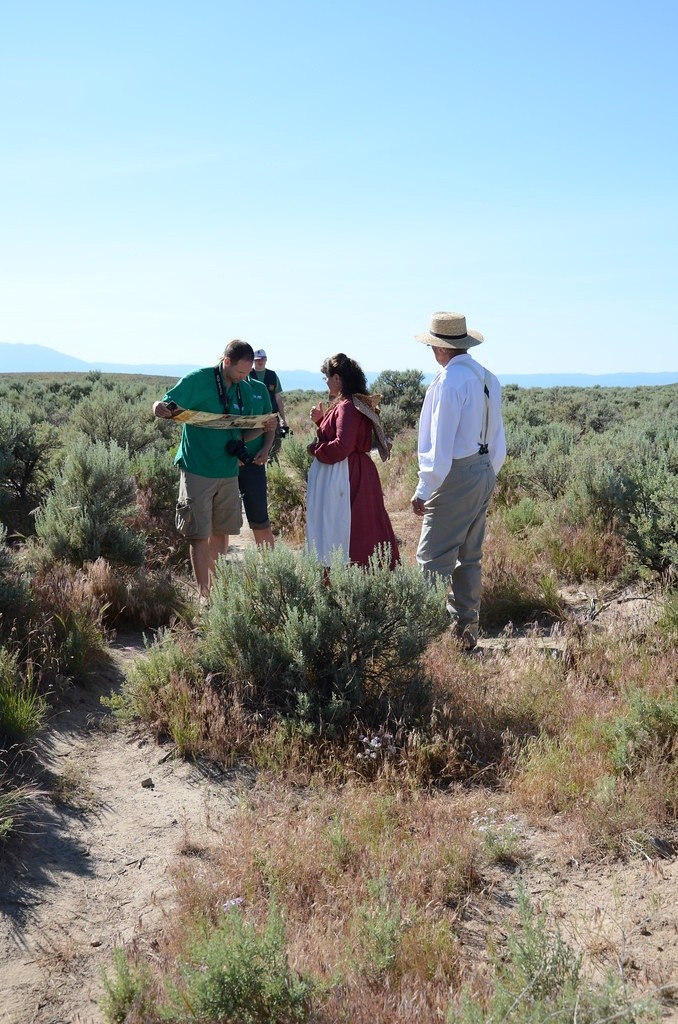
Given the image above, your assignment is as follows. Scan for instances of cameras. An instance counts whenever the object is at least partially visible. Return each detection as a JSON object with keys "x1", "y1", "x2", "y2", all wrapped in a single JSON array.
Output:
[{"x1": 227, "y1": 440, "x2": 254, "y2": 465}]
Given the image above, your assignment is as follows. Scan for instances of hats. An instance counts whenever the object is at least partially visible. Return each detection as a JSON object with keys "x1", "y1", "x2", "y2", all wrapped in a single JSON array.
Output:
[
  {"x1": 254, "y1": 349, "x2": 266, "y2": 360},
  {"x1": 415, "y1": 312, "x2": 484, "y2": 349}
]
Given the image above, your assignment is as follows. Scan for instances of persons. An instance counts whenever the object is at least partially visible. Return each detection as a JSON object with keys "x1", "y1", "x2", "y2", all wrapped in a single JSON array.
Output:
[
  {"x1": 152, "y1": 339, "x2": 279, "y2": 608},
  {"x1": 248, "y1": 349, "x2": 287, "y2": 469},
  {"x1": 305, "y1": 353, "x2": 401, "y2": 593},
  {"x1": 409, "y1": 312, "x2": 506, "y2": 652},
  {"x1": 218, "y1": 353, "x2": 275, "y2": 552}
]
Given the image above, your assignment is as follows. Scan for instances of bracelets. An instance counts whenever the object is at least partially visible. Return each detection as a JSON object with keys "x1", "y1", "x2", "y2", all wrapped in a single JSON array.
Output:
[{"x1": 262, "y1": 427, "x2": 267, "y2": 432}]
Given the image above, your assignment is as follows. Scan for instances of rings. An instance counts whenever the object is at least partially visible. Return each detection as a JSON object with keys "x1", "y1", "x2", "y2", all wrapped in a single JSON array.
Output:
[
  {"x1": 261, "y1": 462, "x2": 263, "y2": 465},
  {"x1": 417, "y1": 509, "x2": 420, "y2": 511}
]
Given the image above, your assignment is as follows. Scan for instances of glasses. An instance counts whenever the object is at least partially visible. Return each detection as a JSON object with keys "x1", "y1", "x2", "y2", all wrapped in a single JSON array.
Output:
[
  {"x1": 427, "y1": 344, "x2": 433, "y2": 350},
  {"x1": 322, "y1": 376, "x2": 332, "y2": 382}
]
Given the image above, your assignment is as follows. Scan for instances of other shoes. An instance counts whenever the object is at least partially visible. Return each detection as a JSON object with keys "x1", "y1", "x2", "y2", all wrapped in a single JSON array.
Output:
[{"x1": 451, "y1": 632, "x2": 478, "y2": 650}]
